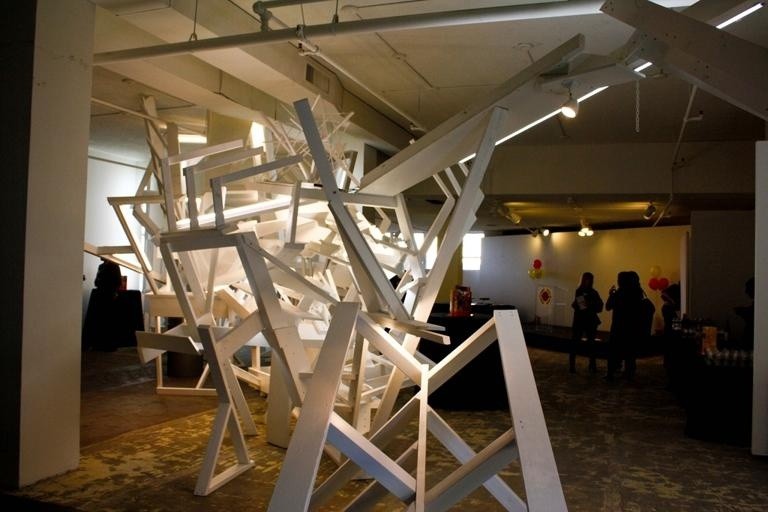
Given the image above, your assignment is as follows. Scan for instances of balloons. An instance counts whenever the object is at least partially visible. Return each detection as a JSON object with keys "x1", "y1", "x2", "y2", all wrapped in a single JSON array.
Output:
[
  {"x1": 532, "y1": 269, "x2": 541, "y2": 279},
  {"x1": 533, "y1": 259, "x2": 541, "y2": 270},
  {"x1": 651, "y1": 264, "x2": 665, "y2": 276},
  {"x1": 528, "y1": 268, "x2": 535, "y2": 279}
]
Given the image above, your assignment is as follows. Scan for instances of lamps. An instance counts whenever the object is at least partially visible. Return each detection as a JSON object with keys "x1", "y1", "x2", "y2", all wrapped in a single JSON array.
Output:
[
  {"x1": 494, "y1": 197, "x2": 657, "y2": 239},
  {"x1": 551, "y1": 77, "x2": 582, "y2": 123}
]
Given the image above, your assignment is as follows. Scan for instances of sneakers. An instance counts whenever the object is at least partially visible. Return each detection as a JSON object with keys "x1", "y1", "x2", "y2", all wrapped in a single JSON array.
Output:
[{"x1": 568, "y1": 367, "x2": 635, "y2": 385}]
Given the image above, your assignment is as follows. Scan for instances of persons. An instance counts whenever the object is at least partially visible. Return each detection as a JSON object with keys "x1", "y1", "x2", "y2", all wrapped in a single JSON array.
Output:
[
  {"x1": 569, "y1": 270, "x2": 682, "y2": 380},
  {"x1": 87, "y1": 252, "x2": 122, "y2": 353},
  {"x1": 734, "y1": 275, "x2": 754, "y2": 350}
]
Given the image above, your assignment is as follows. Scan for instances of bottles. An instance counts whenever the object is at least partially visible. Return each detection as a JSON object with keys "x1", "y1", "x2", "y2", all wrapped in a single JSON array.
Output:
[{"x1": 450, "y1": 285, "x2": 472, "y2": 316}]
